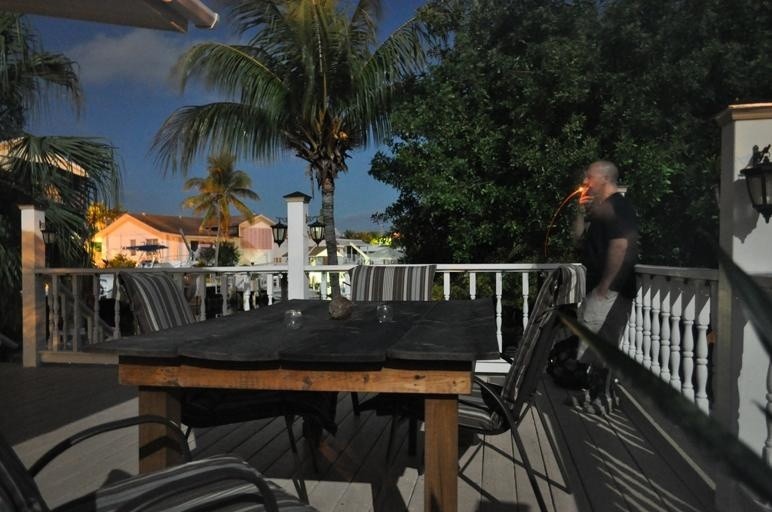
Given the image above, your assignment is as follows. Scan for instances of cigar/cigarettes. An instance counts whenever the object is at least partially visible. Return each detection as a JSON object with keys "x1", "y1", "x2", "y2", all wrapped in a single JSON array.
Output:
[{"x1": 579, "y1": 188, "x2": 587, "y2": 191}]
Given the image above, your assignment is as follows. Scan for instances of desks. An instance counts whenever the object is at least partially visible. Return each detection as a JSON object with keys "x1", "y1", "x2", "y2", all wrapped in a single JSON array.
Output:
[{"x1": 82, "y1": 300, "x2": 498, "y2": 511}]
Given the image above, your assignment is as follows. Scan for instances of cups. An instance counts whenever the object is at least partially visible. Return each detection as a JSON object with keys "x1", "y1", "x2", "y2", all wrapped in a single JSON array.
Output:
[
  {"x1": 284, "y1": 309, "x2": 304, "y2": 329},
  {"x1": 376, "y1": 305, "x2": 393, "y2": 324}
]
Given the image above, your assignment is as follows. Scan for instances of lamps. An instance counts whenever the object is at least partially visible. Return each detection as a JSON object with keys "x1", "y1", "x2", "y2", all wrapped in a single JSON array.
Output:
[
  {"x1": 739, "y1": 144, "x2": 772, "y2": 224},
  {"x1": 268, "y1": 213, "x2": 326, "y2": 249}
]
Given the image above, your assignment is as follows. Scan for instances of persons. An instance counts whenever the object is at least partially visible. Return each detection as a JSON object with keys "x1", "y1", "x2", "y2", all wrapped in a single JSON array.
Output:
[{"x1": 565, "y1": 161, "x2": 631, "y2": 410}]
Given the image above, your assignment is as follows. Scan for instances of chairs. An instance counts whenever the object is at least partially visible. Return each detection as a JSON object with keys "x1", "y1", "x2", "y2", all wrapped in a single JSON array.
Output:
[
  {"x1": 348, "y1": 265, "x2": 438, "y2": 417},
  {"x1": 357, "y1": 266, "x2": 571, "y2": 510},
  {"x1": 3, "y1": 415, "x2": 318, "y2": 511},
  {"x1": 117, "y1": 270, "x2": 340, "y2": 468}
]
